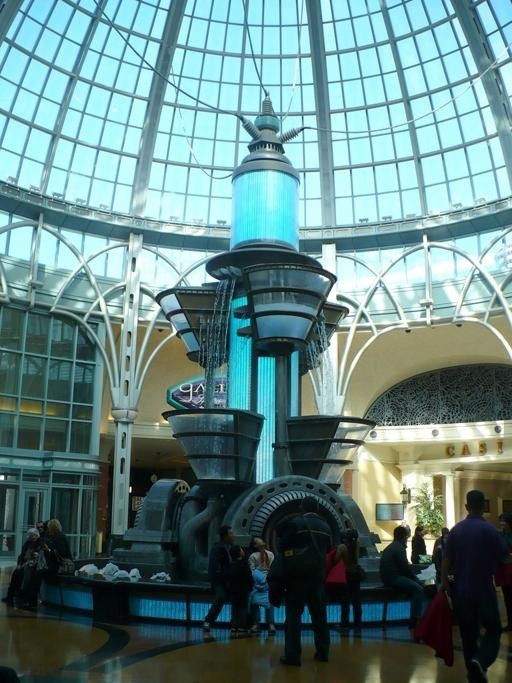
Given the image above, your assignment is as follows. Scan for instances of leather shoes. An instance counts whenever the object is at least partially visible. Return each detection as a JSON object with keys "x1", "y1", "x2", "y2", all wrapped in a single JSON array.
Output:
[
  {"x1": 280, "y1": 656, "x2": 301, "y2": 666},
  {"x1": 315, "y1": 653, "x2": 328, "y2": 662}
]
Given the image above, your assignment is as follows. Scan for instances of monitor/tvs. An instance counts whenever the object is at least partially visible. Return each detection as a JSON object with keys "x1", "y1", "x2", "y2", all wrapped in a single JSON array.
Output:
[{"x1": 376, "y1": 504, "x2": 404, "y2": 520}]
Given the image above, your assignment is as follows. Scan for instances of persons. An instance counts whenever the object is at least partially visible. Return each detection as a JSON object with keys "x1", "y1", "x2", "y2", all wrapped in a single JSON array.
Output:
[
  {"x1": 379, "y1": 526, "x2": 424, "y2": 631},
  {"x1": 411, "y1": 525, "x2": 426, "y2": 564},
  {"x1": 440, "y1": 488, "x2": 510, "y2": 683},
  {"x1": 432, "y1": 527, "x2": 449, "y2": 583},
  {"x1": 201, "y1": 524, "x2": 278, "y2": 634},
  {"x1": 1, "y1": 518, "x2": 73, "y2": 614},
  {"x1": 334, "y1": 528, "x2": 365, "y2": 628},
  {"x1": 493, "y1": 511, "x2": 512, "y2": 631},
  {"x1": 277, "y1": 495, "x2": 334, "y2": 666}
]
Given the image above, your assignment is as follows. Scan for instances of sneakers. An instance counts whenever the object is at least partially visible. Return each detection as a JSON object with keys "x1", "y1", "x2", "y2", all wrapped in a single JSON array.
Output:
[
  {"x1": 202, "y1": 622, "x2": 211, "y2": 631},
  {"x1": 469, "y1": 657, "x2": 489, "y2": 682},
  {"x1": 269, "y1": 623, "x2": 276, "y2": 632},
  {"x1": 2, "y1": 596, "x2": 14, "y2": 605},
  {"x1": 238, "y1": 623, "x2": 248, "y2": 632},
  {"x1": 250, "y1": 625, "x2": 260, "y2": 632},
  {"x1": 231, "y1": 624, "x2": 238, "y2": 631}
]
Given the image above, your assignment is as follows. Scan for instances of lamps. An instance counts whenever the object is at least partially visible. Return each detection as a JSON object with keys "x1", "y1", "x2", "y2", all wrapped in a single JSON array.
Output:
[{"x1": 399, "y1": 484, "x2": 411, "y2": 505}]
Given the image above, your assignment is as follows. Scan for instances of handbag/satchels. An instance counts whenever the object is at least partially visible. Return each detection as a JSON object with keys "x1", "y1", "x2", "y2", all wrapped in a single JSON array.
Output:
[{"x1": 287, "y1": 546, "x2": 324, "y2": 579}]
